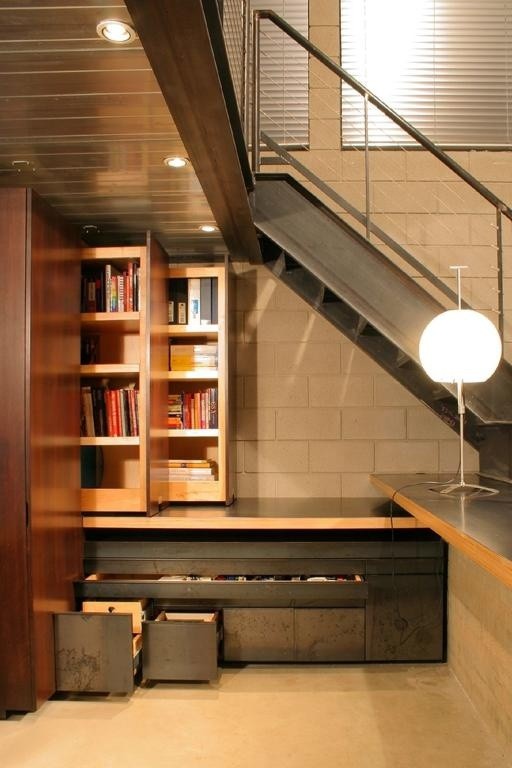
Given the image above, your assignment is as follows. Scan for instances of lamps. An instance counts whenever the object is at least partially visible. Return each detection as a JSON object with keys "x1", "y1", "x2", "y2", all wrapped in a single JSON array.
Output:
[{"x1": 417, "y1": 264, "x2": 505, "y2": 498}]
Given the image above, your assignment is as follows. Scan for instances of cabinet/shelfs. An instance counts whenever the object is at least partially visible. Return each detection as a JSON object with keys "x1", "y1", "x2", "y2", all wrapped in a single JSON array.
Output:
[
  {"x1": 0, "y1": 186, "x2": 84, "y2": 719},
  {"x1": 160, "y1": 250, "x2": 243, "y2": 507},
  {"x1": 38, "y1": 520, "x2": 447, "y2": 700},
  {"x1": 70, "y1": 228, "x2": 175, "y2": 515}
]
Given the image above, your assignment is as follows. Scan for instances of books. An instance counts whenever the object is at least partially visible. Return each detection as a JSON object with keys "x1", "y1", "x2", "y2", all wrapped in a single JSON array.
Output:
[
  {"x1": 167, "y1": 386, "x2": 217, "y2": 429},
  {"x1": 151, "y1": 459, "x2": 218, "y2": 481},
  {"x1": 81, "y1": 260, "x2": 141, "y2": 313},
  {"x1": 80, "y1": 378, "x2": 138, "y2": 436}
]
